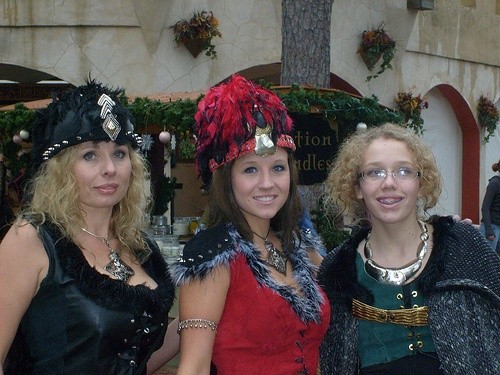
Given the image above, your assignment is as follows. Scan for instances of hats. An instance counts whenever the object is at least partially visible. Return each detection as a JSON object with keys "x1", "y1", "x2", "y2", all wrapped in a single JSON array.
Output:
[
  {"x1": 32, "y1": 70, "x2": 144, "y2": 171},
  {"x1": 191, "y1": 73, "x2": 296, "y2": 179}
]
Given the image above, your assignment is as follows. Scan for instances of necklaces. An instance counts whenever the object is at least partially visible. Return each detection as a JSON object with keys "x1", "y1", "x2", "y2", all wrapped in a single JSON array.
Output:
[
  {"x1": 80, "y1": 225, "x2": 135, "y2": 284},
  {"x1": 252, "y1": 228, "x2": 288, "y2": 277},
  {"x1": 364, "y1": 218, "x2": 430, "y2": 285}
]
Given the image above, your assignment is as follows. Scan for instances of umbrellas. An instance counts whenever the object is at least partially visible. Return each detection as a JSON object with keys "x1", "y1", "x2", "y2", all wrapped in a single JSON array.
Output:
[{"x1": 0, "y1": 98, "x2": 59, "y2": 110}]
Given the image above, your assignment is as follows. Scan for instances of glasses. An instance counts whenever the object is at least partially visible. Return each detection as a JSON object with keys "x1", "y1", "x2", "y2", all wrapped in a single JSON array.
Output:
[{"x1": 358, "y1": 168, "x2": 421, "y2": 182}]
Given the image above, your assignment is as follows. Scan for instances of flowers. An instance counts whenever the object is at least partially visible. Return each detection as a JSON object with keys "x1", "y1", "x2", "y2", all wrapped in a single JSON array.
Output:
[
  {"x1": 355, "y1": 21, "x2": 397, "y2": 81},
  {"x1": 476, "y1": 95, "x2": 500, "y2": 145},
  {"x1": 395, "y1": 89, "x2": 428, "y2": 139},
  {"x1": 171, "y1": 9, "x2": 223, "y2": 60}
]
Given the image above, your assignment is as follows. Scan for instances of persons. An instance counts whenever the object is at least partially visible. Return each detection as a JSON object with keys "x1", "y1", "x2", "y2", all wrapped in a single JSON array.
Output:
[
  {"x1": 315, "y1": 122, "x2": 500, "y2": 375},
  {"x1": 0, "y1": 123, "x2": 36, "y2": 243},
  {"x1": 480, "y1": 160, "x2": 500, "y2": 256},
  {"x1": 0, "y1": 66, "x2": 181, "y2": 375},
  {"x1": 176, "y1": 74, "x2": 330, "y2": 375},
  {"x1": 297, "y1": 188, "x2": 328, "y2": 266}
]
